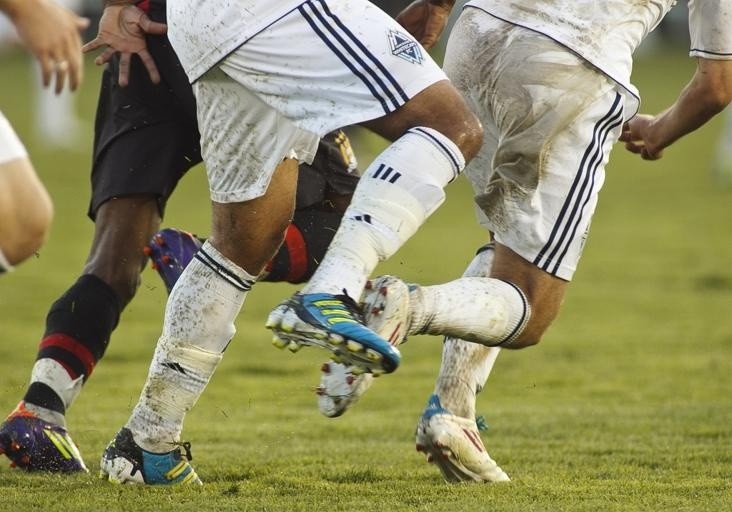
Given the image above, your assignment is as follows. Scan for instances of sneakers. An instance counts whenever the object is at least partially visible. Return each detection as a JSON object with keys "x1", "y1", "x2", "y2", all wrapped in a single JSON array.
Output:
[
  {"x1": 147, "y1": 227, "x2": 206, "y2": 293},
  {"x1": 316, "y1": 274, "x2": 413, "y2": 417},
  {"x1": 97, "y1": 428, "x2": 202, "y2": 487},
  {"x1": 266, "y1": 289, "x2": 402, "y2": 374},
  {"x1": 0, "y1": 404, "x2": 89, "y2": 472},
  {"x1": 415, "y1": 394, "x2": 511, "y2": 484}
]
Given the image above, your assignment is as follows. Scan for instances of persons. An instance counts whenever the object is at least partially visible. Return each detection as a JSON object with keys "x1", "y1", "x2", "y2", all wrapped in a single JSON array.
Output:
[
  {"x1": 1, "y1": 0, "x2": 90, "y2": 276},
  {"x1": 311, "y1": 2, "x2": 732, "y2": 483},
  {"x1": 1, "y1": 0, "x2": 454, "y2": 474},
  {"x1": 98, "y1": 0, "x2": 483, "y2": 490}
]
